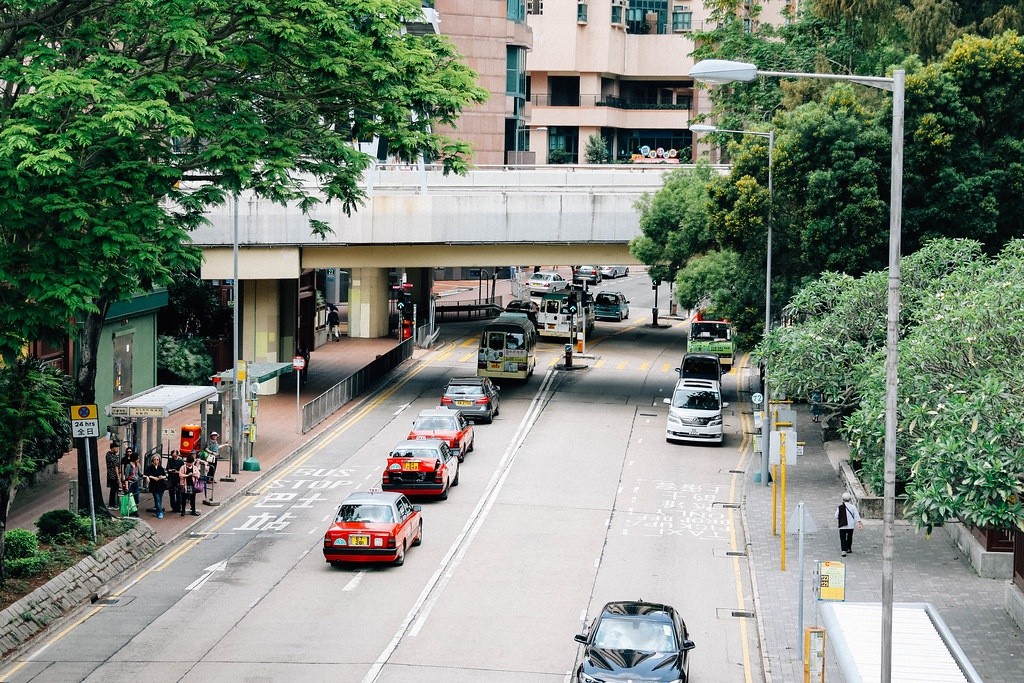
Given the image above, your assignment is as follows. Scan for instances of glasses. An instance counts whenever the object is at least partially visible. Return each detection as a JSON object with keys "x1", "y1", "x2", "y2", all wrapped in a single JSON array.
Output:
[
  {"x1": 212, "y1": 435, "x2": 217, "y2": 436},
  {"x1": 127, "y1": 451, "x2": 131, "y2": 452}
]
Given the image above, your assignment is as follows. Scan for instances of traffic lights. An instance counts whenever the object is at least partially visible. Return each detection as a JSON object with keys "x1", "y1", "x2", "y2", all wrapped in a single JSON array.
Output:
[
  {"x1": 397, "y1": 288, "x2": 404, "y2": 310},
  {"x1": 568, "y1": 292, "x2": 578, "y2": 314},
  {"x1": 581, "y1": 290, "x2": 592, "y2": 308},
  {"x1": 403, "y1": 291, "x2": 412, "y2": 306},
  {"x1": 651, "y1": 279, "x2": 657, "y2": 285}
]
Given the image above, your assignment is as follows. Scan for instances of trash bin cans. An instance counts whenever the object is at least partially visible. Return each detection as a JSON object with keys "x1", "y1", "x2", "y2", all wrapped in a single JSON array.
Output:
[
  {"x1": 179, "y1": 425, "x2": 202, "y2": 457},
  {"x1": 403, "y1": 320, "x2": 412, "y2": 340}
]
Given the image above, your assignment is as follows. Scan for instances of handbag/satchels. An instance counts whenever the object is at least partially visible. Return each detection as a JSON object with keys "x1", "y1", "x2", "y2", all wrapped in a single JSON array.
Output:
[
  {"x1": 206, "y1": 454, "x2": 215, "y2": 463},
  {"x1": 194, "y1": 481, "x2": 203, "y2": 493},
  {"x1": 119, "y1": 492, "x2": 138, "y2": 516}
]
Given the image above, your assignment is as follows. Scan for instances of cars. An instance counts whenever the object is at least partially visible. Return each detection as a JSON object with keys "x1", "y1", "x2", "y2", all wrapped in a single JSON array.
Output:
[
  {"x1": 407, "y1": 406, "x2": 474, "y2": 463},
  {"x1": 600, "y1": 266, "x2": 629, "y2": 279},
  {"x1": 596, "y1": 290, "x2": 631, "y2": 322},
  {"x1": 525, "y1": 270, "x2": 569, "y2": 296},
  {"x1": 663, "y1": 378, "x2": 730, "y2": 447},
  {"x1": 506, "y1": 299, "x2": 540, "y2": 321},
  {"x1": 441, "y1": 376, "x2": 501, "y2": 425},
  {"x1": 322, "y1": 488, "x2": 423, "y2": 568},
  {"x1": 675, "y1": 352, "x2": 727, "y2": 387},
  {"x1": 575, "y1": 597, "x2": 697, "y2": 683},
  {"x1": 382, "y1": 440, "x2": 460, "y2": 501},
  {"x1": 572, "y1": 265, "x2": 602, "y2": 285}
]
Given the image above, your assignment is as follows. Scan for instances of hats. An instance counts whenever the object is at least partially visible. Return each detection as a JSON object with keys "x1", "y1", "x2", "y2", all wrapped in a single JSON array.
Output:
[
  {"x1": 210, "y1": 432, "x2": 219, "y2": 437},
  {"x1": 110, "y1": 444, "x2": 121, "y2": 448},
  {"x1": 842, "y1": 493, "x2": 851, "y2": 501}
]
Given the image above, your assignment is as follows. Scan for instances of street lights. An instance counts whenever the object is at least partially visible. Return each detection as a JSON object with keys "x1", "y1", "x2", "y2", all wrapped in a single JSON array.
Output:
[
  {"x1": 688, "y1": 59, "x2": 906, "y2": 683},
  {"x1": 689, "y1": 123, "x2": 773, "y2": 487}
]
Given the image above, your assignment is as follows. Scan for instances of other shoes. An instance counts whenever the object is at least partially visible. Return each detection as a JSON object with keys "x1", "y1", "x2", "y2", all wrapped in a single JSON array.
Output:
[
  {"x1": 841, "y1": 552, "x2": 846, "y2": 556},
  {"x1": 108, "y1": 507, "x2": 119, "y2": 511},
  {"x1": 181, "y1": 513, "x2": 185, "y2": 516},
  {"x1": 191, "y1": 512, "x2": 199, "y2": 516},
  {"x1": 155, "y1": 513, "x2": 163, "y2": 518},
  {"x1": 847, "y1": 550, "x2": 852, "y2": 553}
]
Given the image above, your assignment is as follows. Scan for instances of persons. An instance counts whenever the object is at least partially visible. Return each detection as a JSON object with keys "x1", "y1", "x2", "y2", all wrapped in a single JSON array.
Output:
[
  {"x1": 326, "y1": 305, "x2": 341, "y2": 342},
  {"x1": 166, "y1": 449, "x2": 210, "y2": 517},
  {"x1": 145, "y1": 453, "x2": 167, "y2": 519},
  {"x1": 120, "y1": 447, "x2": 149, "y2": 518},
  {"x1": 204, "y1": 431, "x2": 229, "y2": 484},
  {"x1": 835, "y1": 492, "x2": 863, "y2": 557},
  {"x1": 106, "y1": 443, "x2": 120, "y2": 510}
]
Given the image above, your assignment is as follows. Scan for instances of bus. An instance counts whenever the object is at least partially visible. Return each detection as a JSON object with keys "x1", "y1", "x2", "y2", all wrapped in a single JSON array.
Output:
[
  {"x1": 686, "y1": 307, "x2": 735, "y2": 372},
  {"x1": 540, "y1": 289, "x2": 595, "y2": 344},
  {"x1": 478, "y1": 316, "x2": 539, "y2": 384}
]
